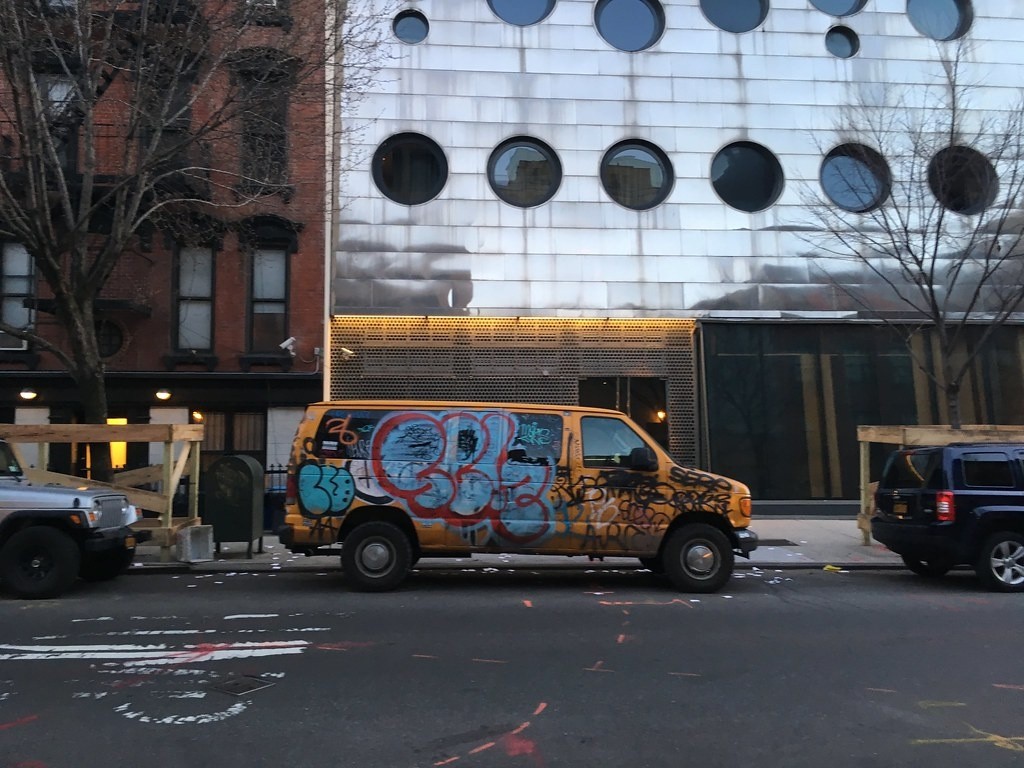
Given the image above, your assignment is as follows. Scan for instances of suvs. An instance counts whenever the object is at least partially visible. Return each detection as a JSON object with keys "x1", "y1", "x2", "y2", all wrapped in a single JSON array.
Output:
[
  {"x1": 0, "y1": 440, "x2": 138, "y2": 601},
  {"x1": 872, "y1": 443, "x2": 1024, "y2": 594}
]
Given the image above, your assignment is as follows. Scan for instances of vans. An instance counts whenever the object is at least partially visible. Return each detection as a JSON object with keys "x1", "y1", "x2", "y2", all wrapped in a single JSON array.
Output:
[{"x1": 280, "y1": 397, "x2": 757, "y2": 596}]
